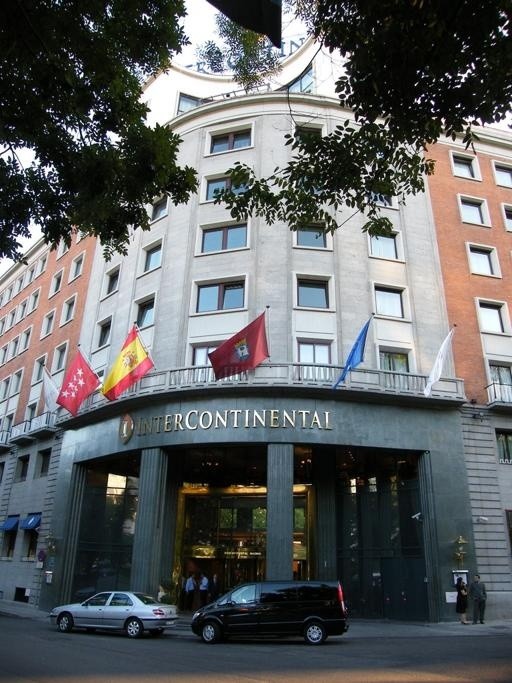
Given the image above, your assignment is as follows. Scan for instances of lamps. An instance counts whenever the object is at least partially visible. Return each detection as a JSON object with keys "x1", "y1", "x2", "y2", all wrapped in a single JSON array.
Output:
[
  {"x1": 44, "y1": 528, "x2": 56, "y2": 552},
  {"x1": 453, "y1": 535, "x2": 468, "y2": 560}
]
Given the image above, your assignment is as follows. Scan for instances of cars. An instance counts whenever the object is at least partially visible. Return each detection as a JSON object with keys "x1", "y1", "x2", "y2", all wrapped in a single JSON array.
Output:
[{"x1": 49, "y1": 589, "x2": 178, "y2": 639}]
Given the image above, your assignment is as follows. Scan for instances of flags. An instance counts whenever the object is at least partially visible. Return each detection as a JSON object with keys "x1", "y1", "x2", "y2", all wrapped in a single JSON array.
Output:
[
  {"x1": 56, "y1": 346, "x2": 102, "y2": 419},
  {"x1": 332, "y1": 317, "x2": 371, "y2": 390},
  {"x1": 207, "y1": 310, "x2": 270, "y2": 381},
  {"x1": 423, "y1": 328, "x2": 456, "y2": 398},
  {"x1": 42, "y1": 365, "x2": 62, "y2": 415},
  {"x1": 98, "y1": 325, "x2": 154, "y2": 401}
]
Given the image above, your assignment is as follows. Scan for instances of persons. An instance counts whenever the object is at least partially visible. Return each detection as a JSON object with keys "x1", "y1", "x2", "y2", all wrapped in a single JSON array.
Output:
[
  {"x1": 469, "y1": 575, "x2": 487, "y2": 624},
  {"x1": 179, "y1": 571, "x2": 220, "y2": 611},
  {"x1": 456, "y1": 577, "x2": 470, "y2": 624}
]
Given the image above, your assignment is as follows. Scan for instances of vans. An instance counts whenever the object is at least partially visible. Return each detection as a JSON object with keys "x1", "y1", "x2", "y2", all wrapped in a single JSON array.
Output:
[{"x1": 190, "y1": 578, "x2": 352, "y2": 647}]
